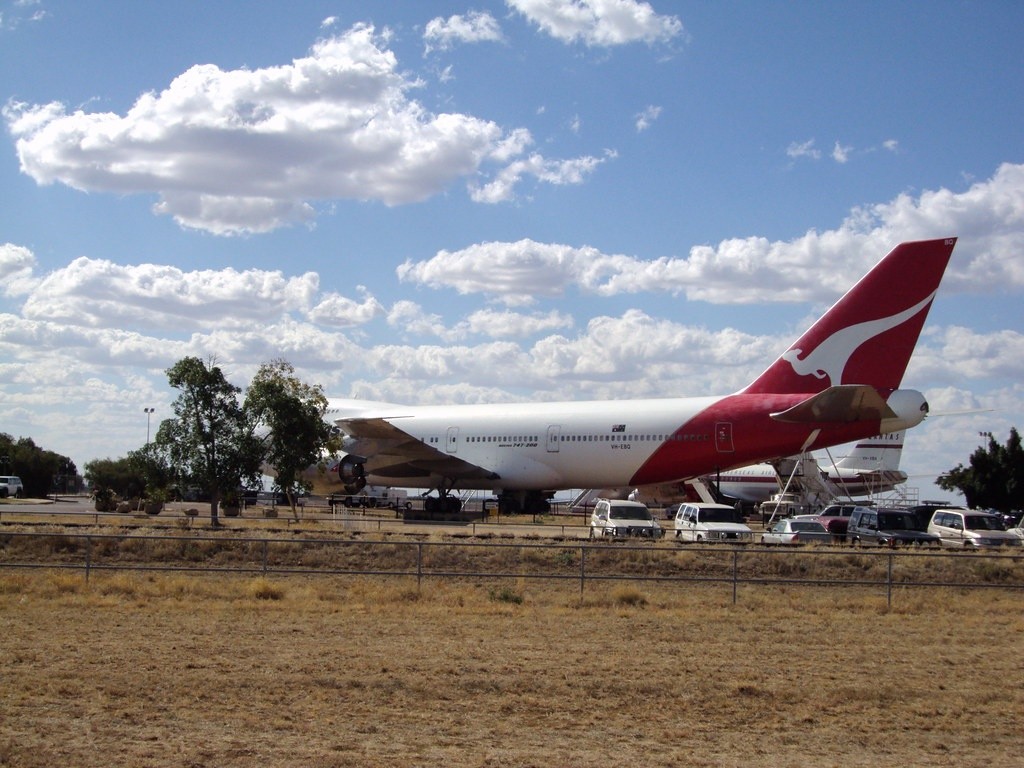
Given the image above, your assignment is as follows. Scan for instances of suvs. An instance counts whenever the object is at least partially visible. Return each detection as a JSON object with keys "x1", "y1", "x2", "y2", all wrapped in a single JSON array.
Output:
[
  {"x1": 846, "y1": 506, "x2": 943, "y2": 548},
  {"x1": 674, "y1": 502, "x2": 752, "y2": 541},
  {"x1": 0, "y1": 474, "x2": 24, "y2": 500},
  {"x1": 924, "y1": 509, "x2": 1023, "y2": 547},
  {"x1": 791, "y1": 503, "x2": 859, "y2": 542},
  {"x1": 589, "y1": 498, "x2": 662, "y2": 540}
]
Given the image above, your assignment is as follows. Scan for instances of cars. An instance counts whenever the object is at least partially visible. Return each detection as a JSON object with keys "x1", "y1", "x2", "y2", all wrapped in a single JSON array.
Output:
[
  {"x1": 760, "y1": 518, "x2": 833, "y2": 546},
  {"x1": 1005, "y1": 516, "x2": 1024, "y2": 547}
]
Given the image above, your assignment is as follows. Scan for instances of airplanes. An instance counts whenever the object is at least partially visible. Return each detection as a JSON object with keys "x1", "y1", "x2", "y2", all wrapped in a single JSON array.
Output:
[{"x1": 243, "y1": 236, "x2": 960, "y2": 514}]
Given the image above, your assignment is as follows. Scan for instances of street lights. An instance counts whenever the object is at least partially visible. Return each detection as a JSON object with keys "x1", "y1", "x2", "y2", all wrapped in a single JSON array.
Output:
[{"x1": 143, "y1": 407, "x2": 156, "y2": 465}]
